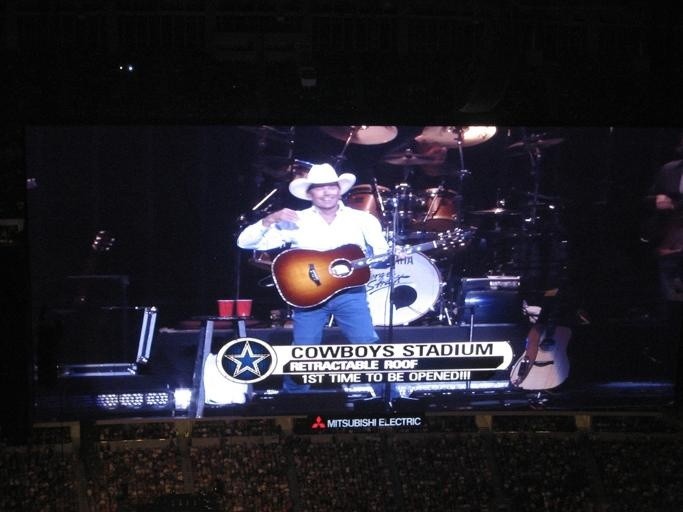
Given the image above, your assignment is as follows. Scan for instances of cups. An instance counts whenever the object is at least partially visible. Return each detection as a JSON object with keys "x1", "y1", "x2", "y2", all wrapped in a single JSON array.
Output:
[
  {"x1": 216, "y1": 299, "x2": 234, "y2": 316},
  {"x1": 233, "y1": 299, "x2": 252, "y2": 317}
]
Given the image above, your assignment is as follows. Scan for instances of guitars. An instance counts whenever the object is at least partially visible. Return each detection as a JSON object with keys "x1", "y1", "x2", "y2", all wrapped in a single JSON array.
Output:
[
  {"x1": 271, "y1": 227, "x2": 471, "y2": 309},
  {"x1": 510, "y1": 287, "x2": 572, "y2": 391}
]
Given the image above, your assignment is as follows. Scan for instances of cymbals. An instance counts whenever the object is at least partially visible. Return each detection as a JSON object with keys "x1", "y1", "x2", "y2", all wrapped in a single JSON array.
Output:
[
  {"x1": 384, "y1": 152, "x2": 434, "y2": 164},
  {"x1": 415, "y1": 126, "x2": 497, "y2": 147},
  {"x1": 506, "y1": 140, "x2": 563, "y2": 155},
  {"x1": 466, "y1": 209, "x2": 525, "y2": 217},
  {"x1": 323, "y1": 126, "x2": 398, "y2": 145}
]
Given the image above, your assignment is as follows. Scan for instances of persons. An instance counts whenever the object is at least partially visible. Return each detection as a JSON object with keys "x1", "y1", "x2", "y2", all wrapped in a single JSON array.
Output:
[
  {"x1": 637, "y1": 143, "x2": 683, "y2": 302},
  {"x1": 234, "y1": 160, "x2": 397, "y2": 405}
]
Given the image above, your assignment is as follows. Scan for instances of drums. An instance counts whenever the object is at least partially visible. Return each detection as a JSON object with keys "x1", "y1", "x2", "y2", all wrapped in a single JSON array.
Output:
[
  {"x1": 411, "y1": 188, "x2": 460, "y2": 230},
  {"x1": 367, "y1": 246, "x2": 442, "y2": 328},
  {"x1": 347, "y1": 184, "x2": 390, "y2": 220}
]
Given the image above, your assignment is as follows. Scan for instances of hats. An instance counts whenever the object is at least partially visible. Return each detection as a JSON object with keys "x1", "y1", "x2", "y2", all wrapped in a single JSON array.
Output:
[{"x1": 289, "y1": 163, "x2": 358, "y2": 201}]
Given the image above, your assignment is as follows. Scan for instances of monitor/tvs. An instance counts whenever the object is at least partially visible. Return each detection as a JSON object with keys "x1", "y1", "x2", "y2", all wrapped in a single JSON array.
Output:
[{"x1": 22, "y1": 115, "x2": 683, "y2": 430}]
